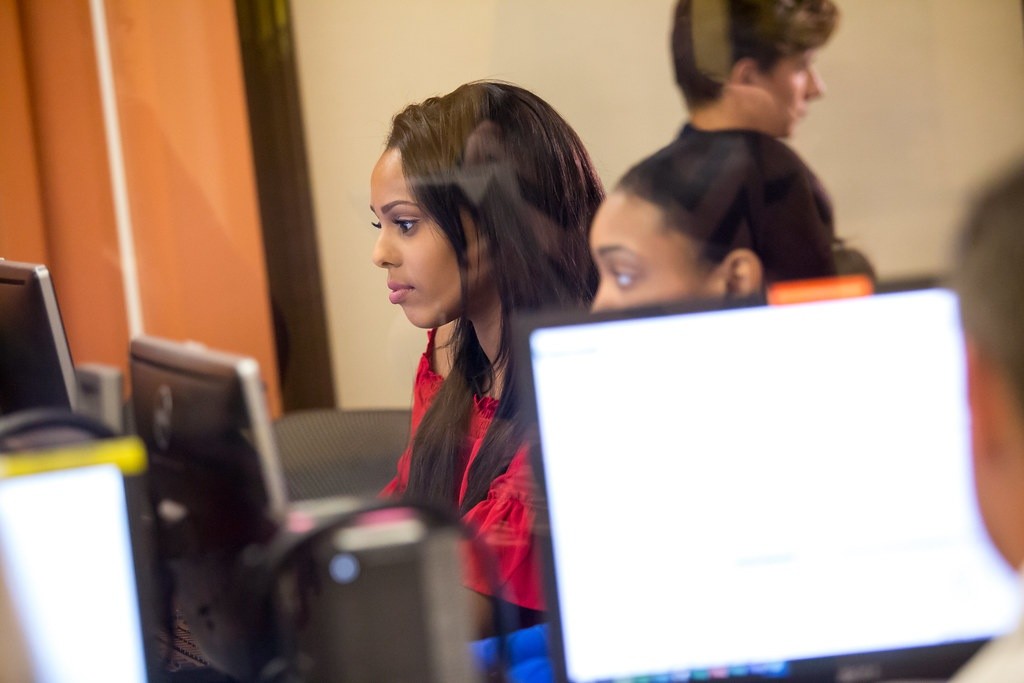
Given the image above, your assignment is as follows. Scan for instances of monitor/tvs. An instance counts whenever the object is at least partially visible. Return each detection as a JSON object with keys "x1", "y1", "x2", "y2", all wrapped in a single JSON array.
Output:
[{"x1": 0, "y1": 259, "x2": 1024, "y2": 682}]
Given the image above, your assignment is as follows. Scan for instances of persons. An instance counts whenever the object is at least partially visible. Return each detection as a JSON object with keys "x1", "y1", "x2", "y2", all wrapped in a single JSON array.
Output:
[
  {"x1": 671, "y1": 0, "x2": 843, "y2": 140},
  {"x1": 364, "y1": 81, "x2": 607, "y2": 641},
  {"x1": 469, "y1": 128, "x2": 886, "y2": 683},
  {"x1": 946, "y1": 167, "x2": 1024, "y2": 683}
]
